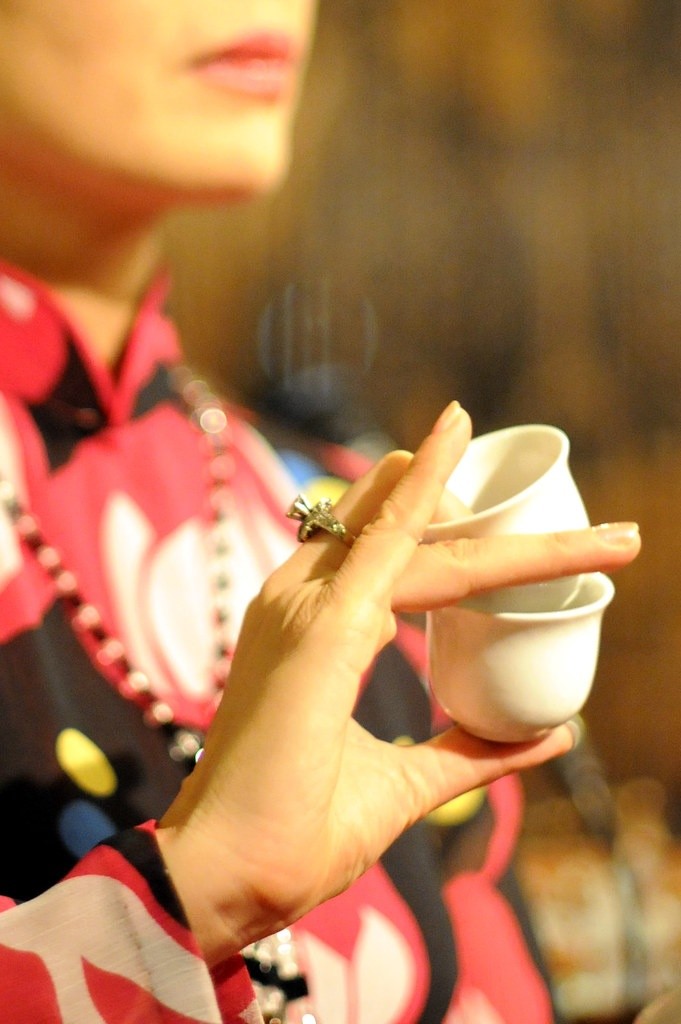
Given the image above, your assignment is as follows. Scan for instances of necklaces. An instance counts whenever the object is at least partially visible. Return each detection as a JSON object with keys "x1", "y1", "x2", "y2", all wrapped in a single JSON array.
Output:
[{"x1": 0, "y1": 362, "x2": 236, "y2": 763}]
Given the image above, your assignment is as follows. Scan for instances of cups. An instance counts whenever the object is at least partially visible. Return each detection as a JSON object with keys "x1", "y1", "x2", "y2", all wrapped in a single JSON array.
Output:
[
  {"x1": 426, "y1": 571, "x2": 618, "y2": 746},
  {"x1": 421, "y1": 424, "x2": 587, "y2": 614}
]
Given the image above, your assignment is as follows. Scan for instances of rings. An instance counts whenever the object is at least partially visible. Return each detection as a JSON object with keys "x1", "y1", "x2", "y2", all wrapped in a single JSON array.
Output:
[{"x1": 286, "y1": 496, "x2": 356, "y2": 547}]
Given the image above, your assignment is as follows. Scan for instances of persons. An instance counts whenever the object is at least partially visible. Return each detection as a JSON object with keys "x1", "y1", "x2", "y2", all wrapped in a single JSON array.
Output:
[{"x1": 0, "y1": 0, "x2": 643, "y2": 1024}]
